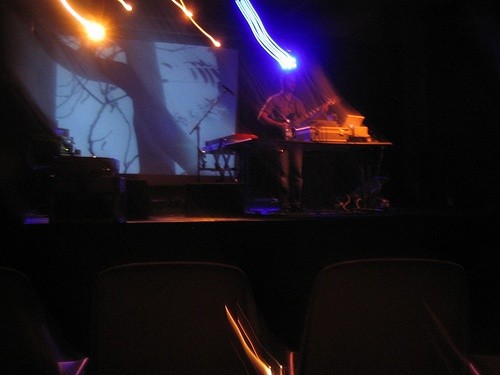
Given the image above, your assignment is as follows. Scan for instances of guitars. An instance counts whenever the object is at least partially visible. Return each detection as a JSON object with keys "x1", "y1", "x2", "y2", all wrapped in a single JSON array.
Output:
[{"x1": 264, "y1": 97, "x2": 338, "y2": 152}]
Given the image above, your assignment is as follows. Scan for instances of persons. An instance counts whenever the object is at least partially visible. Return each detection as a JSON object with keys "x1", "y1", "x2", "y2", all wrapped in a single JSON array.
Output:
[{"x1": 258, "y1": 72, "x2": 307, "y2": 211}]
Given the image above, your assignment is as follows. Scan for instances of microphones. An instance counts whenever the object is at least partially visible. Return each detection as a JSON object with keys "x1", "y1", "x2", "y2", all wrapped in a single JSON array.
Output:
[{"x1": 222, "y1": 85, "x2": 235, "y2": 95}]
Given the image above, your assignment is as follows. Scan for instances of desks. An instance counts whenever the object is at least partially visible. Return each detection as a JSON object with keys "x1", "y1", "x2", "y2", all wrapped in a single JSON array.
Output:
[{"x1": 205, "y1": 136, "x2": 394, "y2": 215}]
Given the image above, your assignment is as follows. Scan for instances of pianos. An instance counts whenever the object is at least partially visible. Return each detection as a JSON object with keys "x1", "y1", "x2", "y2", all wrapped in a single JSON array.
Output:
[{"x1": 203, "y1": 134, "x2": 261, "y2": 183}]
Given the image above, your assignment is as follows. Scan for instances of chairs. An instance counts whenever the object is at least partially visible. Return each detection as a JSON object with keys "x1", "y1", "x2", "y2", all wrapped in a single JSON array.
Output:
[
  {"x1": 289, "y1": 257, "x2": 500, "y2": 375},
  {"x1": 90, "y1": 261, "x2": 286, "y2": 375},
  {"x1": 0, "y1": 265, "x2": 89, "y2": 375}
]
